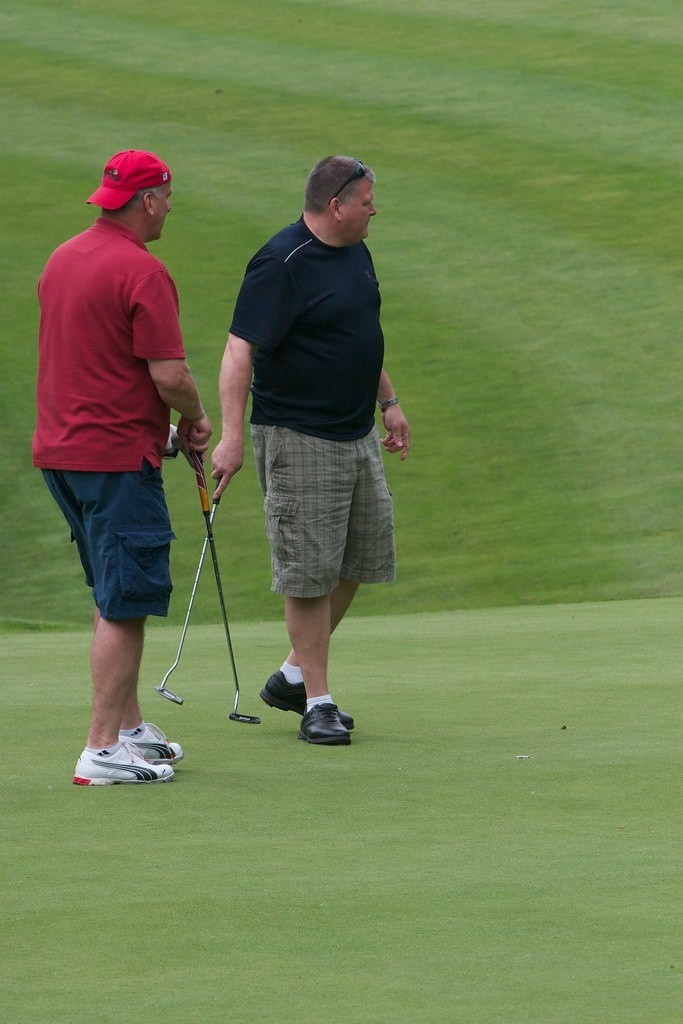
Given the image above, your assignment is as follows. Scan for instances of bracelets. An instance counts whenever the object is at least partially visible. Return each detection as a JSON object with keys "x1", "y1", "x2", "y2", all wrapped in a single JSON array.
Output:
[{"x1": 376, "y1": 397, "x2": 399, "y2": 412}]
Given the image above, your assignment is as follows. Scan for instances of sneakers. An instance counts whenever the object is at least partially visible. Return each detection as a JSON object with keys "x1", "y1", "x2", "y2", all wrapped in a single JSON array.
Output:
[
  {"x1": 73, "y1": 740, "x2": 177, "y2": 784},
  {"x1": 118, "y1": 722, "x2": 185, "y2": 763}
]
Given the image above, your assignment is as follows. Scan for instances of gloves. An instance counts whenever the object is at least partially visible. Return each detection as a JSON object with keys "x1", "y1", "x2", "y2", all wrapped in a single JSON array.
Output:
[{"x1": 164, "y1": 423, "x2": 181, "y2": 460}]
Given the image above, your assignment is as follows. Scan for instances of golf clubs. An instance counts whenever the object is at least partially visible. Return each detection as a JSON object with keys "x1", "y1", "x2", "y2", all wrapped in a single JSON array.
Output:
[
  {"x1": 192, "y1": 451, "x2": 261, "y2": 725},
  {"x1": 154, "y1": 474, "x2": 221, "y2": 706}
]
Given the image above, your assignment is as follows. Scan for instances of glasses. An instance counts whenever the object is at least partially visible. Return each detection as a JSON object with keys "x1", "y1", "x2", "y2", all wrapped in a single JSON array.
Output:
[{"x1": 328, "y1": 159, "x2": 365, "y2": 204}]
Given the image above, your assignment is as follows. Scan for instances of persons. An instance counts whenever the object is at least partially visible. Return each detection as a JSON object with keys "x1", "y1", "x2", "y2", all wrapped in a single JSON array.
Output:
[
  {"x1": 210, "y1": 155, "x2": 411, "y2": 744},
  {"x1": 31, "y1": 148, "x2": 213, "y2": 786}
]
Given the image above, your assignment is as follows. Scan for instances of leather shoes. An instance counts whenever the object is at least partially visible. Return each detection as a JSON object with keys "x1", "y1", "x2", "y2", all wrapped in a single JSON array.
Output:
[
  {"x1": 259, "y1": 669, "x2": 354, "y2": 729},
  {"x1": 298, "y1": 703, "x2": 351, "y2": 745}
]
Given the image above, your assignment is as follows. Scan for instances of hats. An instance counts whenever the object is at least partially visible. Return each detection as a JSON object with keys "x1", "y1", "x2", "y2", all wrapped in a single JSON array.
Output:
[{"x1": 86, "y1": 150, "x2": 171, "y2": 209}]
